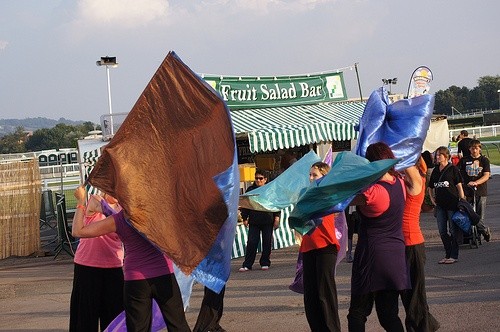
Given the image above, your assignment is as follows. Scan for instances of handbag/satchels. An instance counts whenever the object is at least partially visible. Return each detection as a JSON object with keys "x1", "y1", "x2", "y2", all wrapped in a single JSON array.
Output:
[
  {"x1": 433, "y1": 205, "x2": 437, "y2": 217},
  {"x1": 452, "y1": 211, "x2": 471, "y2": 234},
  {"x1": 447, "y1": 221, "x2": 463, "y2": 237}
]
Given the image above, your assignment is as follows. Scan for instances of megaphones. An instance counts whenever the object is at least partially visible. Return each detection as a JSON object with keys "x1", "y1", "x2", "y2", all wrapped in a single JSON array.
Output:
[{"x1": 101, "y1": 57, "x2": 116, "y2": 65}]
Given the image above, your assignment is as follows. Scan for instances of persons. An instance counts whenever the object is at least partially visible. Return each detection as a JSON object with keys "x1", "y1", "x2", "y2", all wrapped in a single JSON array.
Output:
[
  {"x1": 347, "y1": 142, "x2": 412, "y2": 332},
  {"x1": 458, "y1": 140, "x2": 490, "y2": 245},
  {"x1": 69, "y1": 187, "x2": 126, "y2": 332},
  {"x1": 429, "y1": 146, "x2": 464, "y2": 264},
  {"x1": 299, "y1": 162, "x2": 341, "y2": 332},
  {"x1": 237, "y1": 170, "x2": 281, "y2": 272},
  {"x1": 458, "y1": 130, "x2": 472, "y2": 159},
  {"x1": 400, "y1": 147, "x2": 440, "y2": 332},
  {"x1": 72, "y1": 185, "x2": 192, "y2": 332}
]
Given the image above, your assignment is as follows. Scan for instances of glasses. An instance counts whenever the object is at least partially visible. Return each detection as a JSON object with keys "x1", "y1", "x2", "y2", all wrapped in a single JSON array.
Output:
[{"x1": 255, "y1": 177, "x2": 264, "y2": 180}]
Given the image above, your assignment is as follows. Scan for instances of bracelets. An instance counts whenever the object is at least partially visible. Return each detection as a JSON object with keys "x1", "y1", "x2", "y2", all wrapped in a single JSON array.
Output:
[{"x1": 77, "y1": 204, "x2": 86, "y2": 212}]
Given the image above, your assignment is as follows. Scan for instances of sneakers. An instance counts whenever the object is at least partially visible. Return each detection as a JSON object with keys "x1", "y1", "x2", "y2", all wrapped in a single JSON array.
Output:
[{"x1": 482, "y1": 227, "x2": 491, "y2": 242}]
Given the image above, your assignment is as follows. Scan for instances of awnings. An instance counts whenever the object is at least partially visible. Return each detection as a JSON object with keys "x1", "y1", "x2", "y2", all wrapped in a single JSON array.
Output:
[{"x1": 229, "y1": 101, "x2": 367, "y2": 153}]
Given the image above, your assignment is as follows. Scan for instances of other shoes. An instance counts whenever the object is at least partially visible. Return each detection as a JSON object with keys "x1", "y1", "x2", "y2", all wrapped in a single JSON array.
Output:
[
  {"x1": 261, "y1": 266, "x2": 268, "y2": 270},
  {"x1": 239, "y1": 267, "x2": 248, "y2": 271}
]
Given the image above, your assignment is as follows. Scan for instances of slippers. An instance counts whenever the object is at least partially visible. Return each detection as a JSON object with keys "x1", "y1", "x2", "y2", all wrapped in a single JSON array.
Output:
[
  {"x1": 445, "y1": 260, "x2": 457, "y2": 264},
  {"x1": 438, "y1": 260, "x2": 444, "y2": 263}
]
